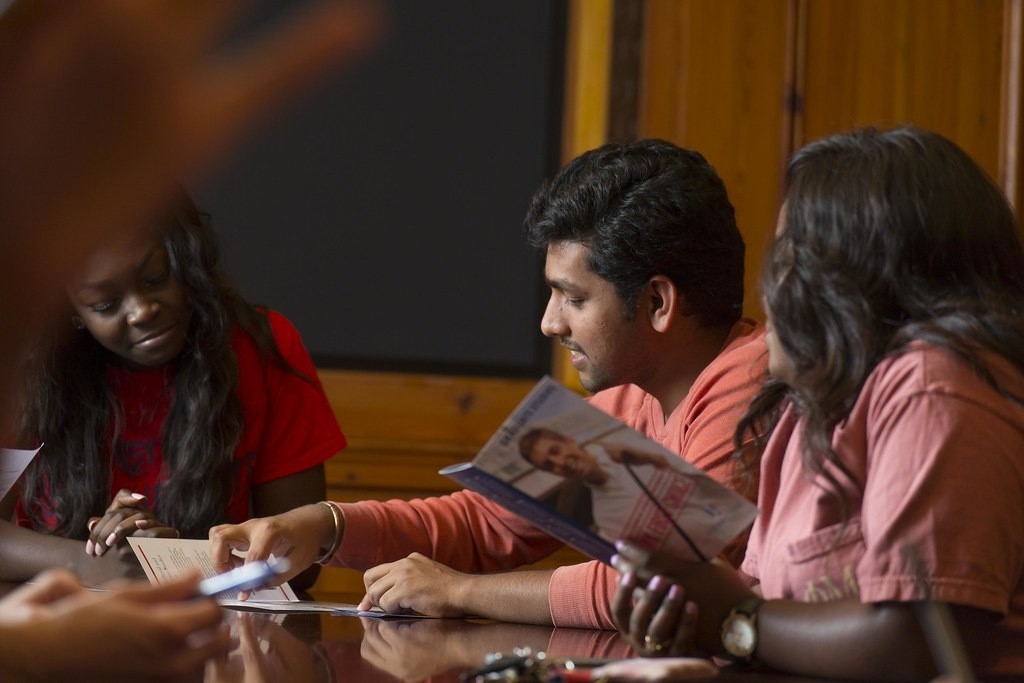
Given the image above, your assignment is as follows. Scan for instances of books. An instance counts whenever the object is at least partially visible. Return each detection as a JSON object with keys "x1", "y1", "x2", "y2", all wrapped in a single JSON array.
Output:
[{"x1": 438, "y1": 374, "x2": 759, "y2": 577}]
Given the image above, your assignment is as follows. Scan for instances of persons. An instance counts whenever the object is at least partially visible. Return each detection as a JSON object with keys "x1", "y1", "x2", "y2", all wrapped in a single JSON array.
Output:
[
  {"x1": 610, "y1": 125, "x2": 1024, "y2": 683},
  {"x1": 0, "y1": 1, "x2": 379, "y2": 683},
  {"x1": 209, "y1": 135, "x2": 785, "y2": 629},
  {"x1": 519, "y1": 426, "x2": 757, "y2": 564}
]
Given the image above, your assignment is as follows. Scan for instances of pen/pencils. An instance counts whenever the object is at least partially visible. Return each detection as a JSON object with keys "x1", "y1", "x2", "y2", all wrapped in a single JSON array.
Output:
[{"x1": 190, "y1": 557, "x2": 294, "y2": 597}]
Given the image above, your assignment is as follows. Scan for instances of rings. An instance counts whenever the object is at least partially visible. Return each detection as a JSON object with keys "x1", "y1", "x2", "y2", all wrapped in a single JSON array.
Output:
[
  {"x1": 175, "y1": 529, "x2": 180, "y2": 538},
  {"x1": 643, "y1": 635, "x2": 670, "y2": 651}
]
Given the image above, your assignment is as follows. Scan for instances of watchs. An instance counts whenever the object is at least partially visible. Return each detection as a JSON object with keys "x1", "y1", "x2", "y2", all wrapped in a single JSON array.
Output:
[{"x1": 721, "y1": 596, "x2": 768, "y2": 659}]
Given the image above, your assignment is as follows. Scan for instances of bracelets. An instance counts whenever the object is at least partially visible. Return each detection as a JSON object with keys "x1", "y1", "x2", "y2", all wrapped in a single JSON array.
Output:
[{"x1": 313, "y1": 501, "x2": 340, "y2": 563}]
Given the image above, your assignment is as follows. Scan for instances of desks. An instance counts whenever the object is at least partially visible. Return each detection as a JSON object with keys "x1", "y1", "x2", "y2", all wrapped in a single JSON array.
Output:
[{"x1": 0, "y1": 567, "x2": 841, "y2": 683}]
